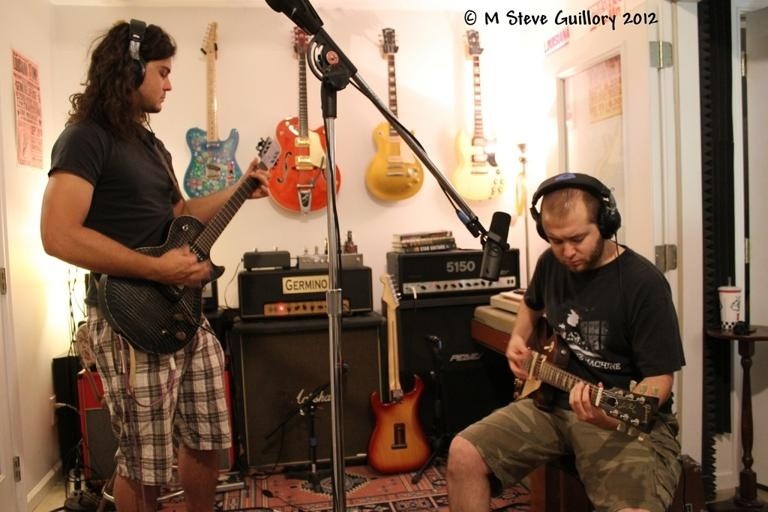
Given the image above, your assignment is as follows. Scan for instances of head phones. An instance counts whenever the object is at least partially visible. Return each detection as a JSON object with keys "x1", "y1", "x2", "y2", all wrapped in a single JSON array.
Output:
[
  {"x1": 529, "y1": 172, "x2": 621, "y2": 243},
  {"x1": 128, "y1": 19, "x2": 147, "y2": 89}
]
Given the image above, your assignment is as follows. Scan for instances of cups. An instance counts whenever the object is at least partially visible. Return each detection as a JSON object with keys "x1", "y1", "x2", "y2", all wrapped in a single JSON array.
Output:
[{"x1": 717, "y1": 286, "x2": 743, "y2": 330}]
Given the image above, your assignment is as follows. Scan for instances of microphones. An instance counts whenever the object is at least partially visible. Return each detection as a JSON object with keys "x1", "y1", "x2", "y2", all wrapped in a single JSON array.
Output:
[{"x1": 479, "y1": 211, "x2": 511, "y2": 281}]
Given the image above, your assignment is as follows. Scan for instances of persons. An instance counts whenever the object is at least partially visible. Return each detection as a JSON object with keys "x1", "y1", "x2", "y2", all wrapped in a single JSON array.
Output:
[
  {"x1": 39, "y1": 18, "x2": 272, "y2": 512},
  {"x1": 445, "y1": 172, "x2": 684, "y2": 512}
]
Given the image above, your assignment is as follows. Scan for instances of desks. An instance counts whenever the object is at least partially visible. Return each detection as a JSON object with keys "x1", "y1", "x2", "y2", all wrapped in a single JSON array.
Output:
[{"x1": 705, "y1": 324, "x2": 766, "y2": 511}]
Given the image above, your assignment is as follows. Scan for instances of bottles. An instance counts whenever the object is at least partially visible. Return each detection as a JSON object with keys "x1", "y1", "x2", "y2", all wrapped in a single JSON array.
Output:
[{"x1": 344, "y1": 230, "x2": 357, "y2": 254}]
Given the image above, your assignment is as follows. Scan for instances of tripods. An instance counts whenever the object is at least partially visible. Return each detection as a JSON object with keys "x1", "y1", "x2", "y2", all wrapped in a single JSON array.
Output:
[
  {"x1": 266, "y1": 363, "x2": 350, "y2": 492},
  {"x1": 411, "y1": 335, "x2": 449, "y2": 484}
]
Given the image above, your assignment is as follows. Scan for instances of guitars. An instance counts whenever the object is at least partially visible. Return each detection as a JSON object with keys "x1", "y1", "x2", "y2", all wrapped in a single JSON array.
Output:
[
  {"x1": 452, "y1": 28, "x2": 504, "y2": 205},
  {"x1": 366, "y1": 28, "x2": 423, "y2": 203},
  {"x1": 183, "y1": 22, "x2": 244, "y2": 198},
  {"x1": 268, "y1": 26, "x2": 340, "y2": 210},
  {"x1": 368, "y1": 273, "x2": 431, "y2": 471},
  {"x1": 510, "y1": 333, "x2": 661, "y2": 443},
  {"x1": 97, "y1": 137, "x2": 282, "y2": 356}
]
